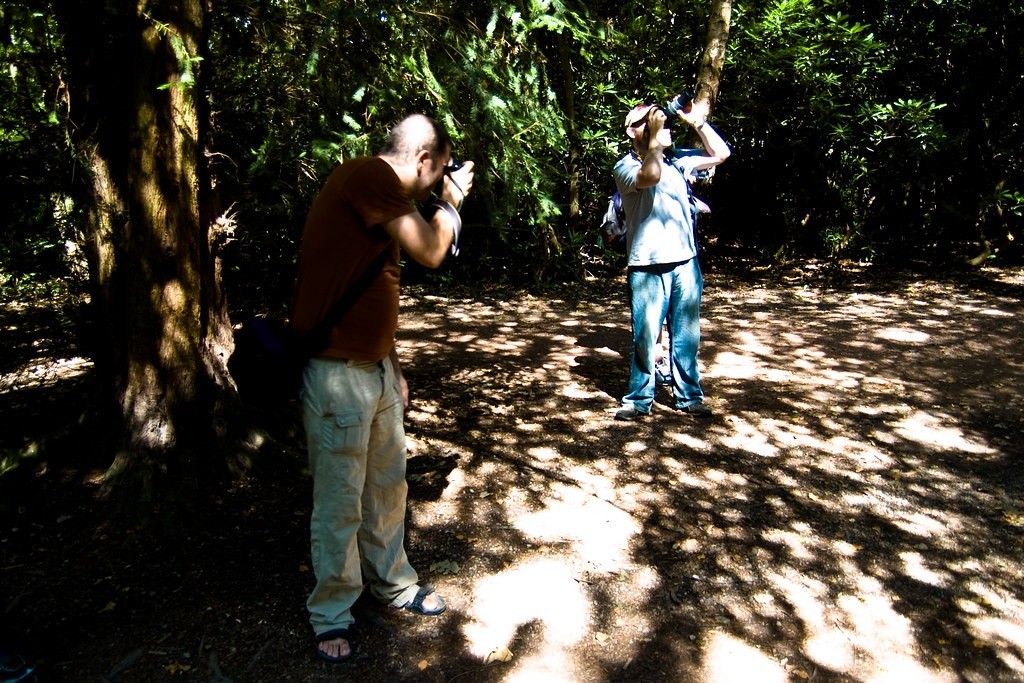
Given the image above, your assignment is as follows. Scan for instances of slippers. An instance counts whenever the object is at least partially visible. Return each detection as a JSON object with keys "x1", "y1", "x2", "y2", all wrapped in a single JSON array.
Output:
[
  {"x1": 314, "y1": 628, "x2": 354, "y2": 663},
  {"x1": 404, "y1": 586, "x2": 447, "y2": 615}
]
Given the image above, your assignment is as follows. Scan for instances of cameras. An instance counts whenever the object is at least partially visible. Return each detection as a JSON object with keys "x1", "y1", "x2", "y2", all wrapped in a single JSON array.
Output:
[
  {"x1": 434, "y1": 163, "x2": 464, "y2": 196},
  {"x1": 646, "y1": 91, "x2": 694, "y2": 130}
]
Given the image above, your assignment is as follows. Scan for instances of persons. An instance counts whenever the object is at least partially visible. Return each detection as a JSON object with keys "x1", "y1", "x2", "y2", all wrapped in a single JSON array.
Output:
[
  {"x1": 613, "y1": 100, "x2": 731, "y2": 420},
  {"x1": 292, "y1": 113, "x2": 475, "y2": 663}
]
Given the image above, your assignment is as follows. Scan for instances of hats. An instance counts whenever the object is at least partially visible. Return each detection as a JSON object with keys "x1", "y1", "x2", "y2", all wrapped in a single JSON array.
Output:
[{"x1": 625, "y1": 104, "x2": 657, "y2": 127}]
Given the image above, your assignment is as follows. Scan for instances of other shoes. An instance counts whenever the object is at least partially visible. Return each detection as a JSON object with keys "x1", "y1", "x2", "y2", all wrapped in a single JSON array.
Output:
[
  {"x1": 683, "y1": 401, "x2": 713, "y2": 417},
  {"x1": 616, "y1": 405, "x2": 641, "y2": 420}
]
